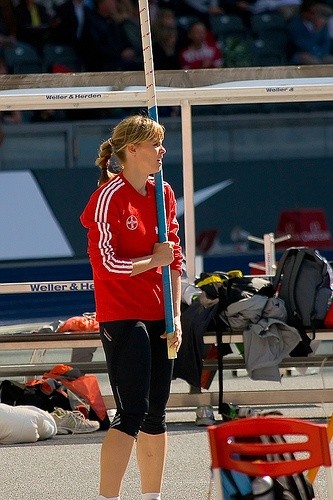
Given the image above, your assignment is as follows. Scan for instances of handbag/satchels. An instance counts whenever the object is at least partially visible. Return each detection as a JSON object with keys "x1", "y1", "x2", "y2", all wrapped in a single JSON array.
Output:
[{"x1": 27, "y1": 365, "x2": 109, "y2": 431}]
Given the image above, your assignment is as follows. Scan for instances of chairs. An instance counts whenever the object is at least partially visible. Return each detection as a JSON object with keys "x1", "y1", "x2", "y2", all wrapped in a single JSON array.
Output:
[{"x1": 208, "y1": 419, "x2": 333, "y2": 500}]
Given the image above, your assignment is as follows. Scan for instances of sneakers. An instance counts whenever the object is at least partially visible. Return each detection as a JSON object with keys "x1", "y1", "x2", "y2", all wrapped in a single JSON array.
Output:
[
  {"x1": 49, "y1": 409, "x2": 100, "y2": 436},
  {"x1": 194, "y1": 403, "x2": 214, "y2": 426}
]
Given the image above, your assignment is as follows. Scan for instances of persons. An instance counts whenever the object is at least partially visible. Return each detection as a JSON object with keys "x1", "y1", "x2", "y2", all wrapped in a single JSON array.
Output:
[
  {"x1": 1, "y1": 0, "x2": 333, "y2": 168},
  {"x1": 79, "y1": 113, "x2": 185, "y2": 499}
]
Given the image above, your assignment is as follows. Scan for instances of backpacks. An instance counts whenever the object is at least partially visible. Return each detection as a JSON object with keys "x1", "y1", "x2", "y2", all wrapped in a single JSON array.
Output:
[
  {"x1": 269, "y1": 245, "x2": 333, "y2": 341},
  {"x1": 221, "y1": 409, "x2": 316, "y2": 500}
]
